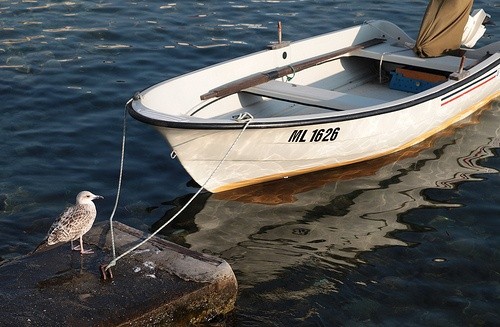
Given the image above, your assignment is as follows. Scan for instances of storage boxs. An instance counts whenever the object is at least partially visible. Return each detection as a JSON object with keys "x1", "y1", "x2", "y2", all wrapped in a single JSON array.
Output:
[{"x1": 389, "y1": 65, "x2": 446, "y2": 94}]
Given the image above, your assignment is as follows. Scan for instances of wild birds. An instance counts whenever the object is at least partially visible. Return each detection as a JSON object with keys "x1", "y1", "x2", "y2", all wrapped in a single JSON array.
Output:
[{"x1": 30, "y1": 191, "x2": 105, "y2": 255}]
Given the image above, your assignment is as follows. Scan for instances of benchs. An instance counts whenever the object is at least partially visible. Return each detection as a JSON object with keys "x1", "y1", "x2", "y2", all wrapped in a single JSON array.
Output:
[
  {"x1": 241, "y1": 79, "x2": 387, "y2": 110},
  {"x1": 356, "y1": 44, "x2": 478, "y2": 73}
]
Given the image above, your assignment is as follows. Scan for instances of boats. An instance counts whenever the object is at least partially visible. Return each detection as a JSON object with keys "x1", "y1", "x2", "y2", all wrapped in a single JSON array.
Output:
[{"x1": 126, "y1": 0, "x2": 500, "y2": 194}]
